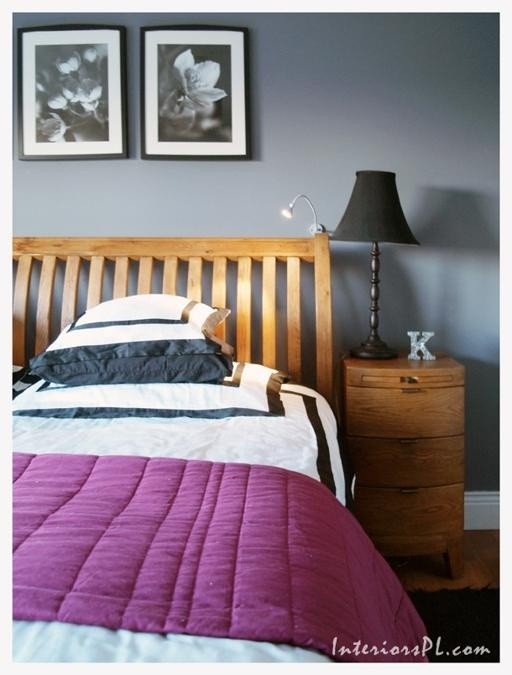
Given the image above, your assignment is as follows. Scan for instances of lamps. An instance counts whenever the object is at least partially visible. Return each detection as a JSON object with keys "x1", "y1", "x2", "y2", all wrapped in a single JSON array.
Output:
[
  {"x1": 329, "y1": 170, "x2": 420, "y2": 361},
  {"x1": 282, "y1": 195, "x2": 324, "y2": 236}
]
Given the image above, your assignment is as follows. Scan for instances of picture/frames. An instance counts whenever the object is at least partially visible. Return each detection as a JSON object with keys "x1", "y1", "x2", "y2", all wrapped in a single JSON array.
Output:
[
  {"x1": 140, "y1": 25, "x2": 253, "y2": 160},
  {"x1": 17, "y1": 25, "x2": 131, "y2": 160}
]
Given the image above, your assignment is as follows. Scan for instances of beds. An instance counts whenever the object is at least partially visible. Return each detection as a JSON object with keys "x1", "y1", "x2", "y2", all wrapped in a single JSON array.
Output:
[{"x1": 13, "y1": 234, "x2": 430, "y2": 662}]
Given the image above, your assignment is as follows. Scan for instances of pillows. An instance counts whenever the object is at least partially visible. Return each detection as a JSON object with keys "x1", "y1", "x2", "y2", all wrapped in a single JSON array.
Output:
[{"x1": 13, "y1": 293, "x2": 288, "y2": 417}]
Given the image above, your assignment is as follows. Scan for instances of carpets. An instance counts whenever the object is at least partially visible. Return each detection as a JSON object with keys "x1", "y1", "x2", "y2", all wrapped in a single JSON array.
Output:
[{"x1": 409, "y1": 586, "x2": 499, "y2": 662}]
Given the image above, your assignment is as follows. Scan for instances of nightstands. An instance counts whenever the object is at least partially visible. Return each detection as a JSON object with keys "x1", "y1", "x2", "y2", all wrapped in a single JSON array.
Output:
[{"x1": 341, "y1": 350, "x2": 465, "y2": 579}]
